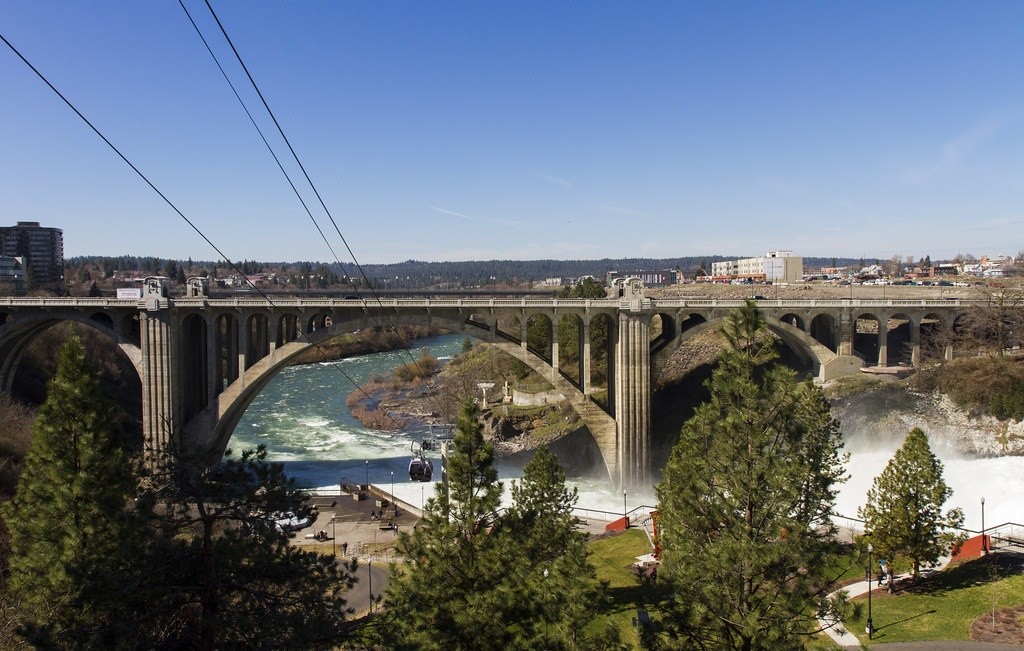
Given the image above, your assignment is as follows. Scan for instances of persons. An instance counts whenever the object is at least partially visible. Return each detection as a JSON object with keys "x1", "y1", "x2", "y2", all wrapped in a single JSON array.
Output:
[
  {"x1": 876, "y1": 565, "x2": 883, "y2": 586},
  {"x1": 370, "y1": 508, "x2": 383, "y2": 521},
  {"x1": 864, "y1": 560, "x2": 871, "y2": 581},
  {"x1": 342, "y1": 542, "x2": 348, "y2": 556},
  {"x1": 394, "y1": 523, "x2": 398, "y2": 535}
]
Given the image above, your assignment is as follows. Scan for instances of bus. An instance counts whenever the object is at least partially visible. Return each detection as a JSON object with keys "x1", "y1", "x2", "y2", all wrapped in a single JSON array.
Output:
[{"x1": 823, "y1": 274, "x2": 841, "y2": 279}]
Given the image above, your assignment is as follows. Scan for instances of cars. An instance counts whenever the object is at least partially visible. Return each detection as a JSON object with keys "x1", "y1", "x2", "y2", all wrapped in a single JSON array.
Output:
[
  {"x1": 937, "y1": 280, "x2": 954, "y2": 286},
  {"x1": 967, "y1": 281, "x2": 988, "y2": 288},
  {"x1": 892, "y1": 280, "x2": 935, "y2": 286},
  {"x1": 731, "y1": 279, "x2": 772, "y2": 285},
  {"x1": 841, "y1": 279, "x2": 892, "y2": 285}
]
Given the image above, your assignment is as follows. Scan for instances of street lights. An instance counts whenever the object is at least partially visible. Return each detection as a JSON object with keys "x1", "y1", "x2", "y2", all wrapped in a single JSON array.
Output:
[
  {"x1": 624, "y1": 490, "x2": 627, "y2": 529},
  {"x1": 332, "y1": 515, "x2": 336, "y2": 556},
  {"x1": 391, "y1": 471, "x2": 393, "y2": 503},
  {"x1": 365, "y1": 459, "x2": 368, "y2": 490},
  {"x1": 868, "y1": 543, "x2": 874, "y2": 639},
  {"x1": 368, "y1": 556, "x2": 372, "y2": 613},
  {"x1": 422, "y1": 485, "x2": 424, "y2": 518},
  {"x1": 981, "y1": 497, "x2": 985, "y2": 550}
]
